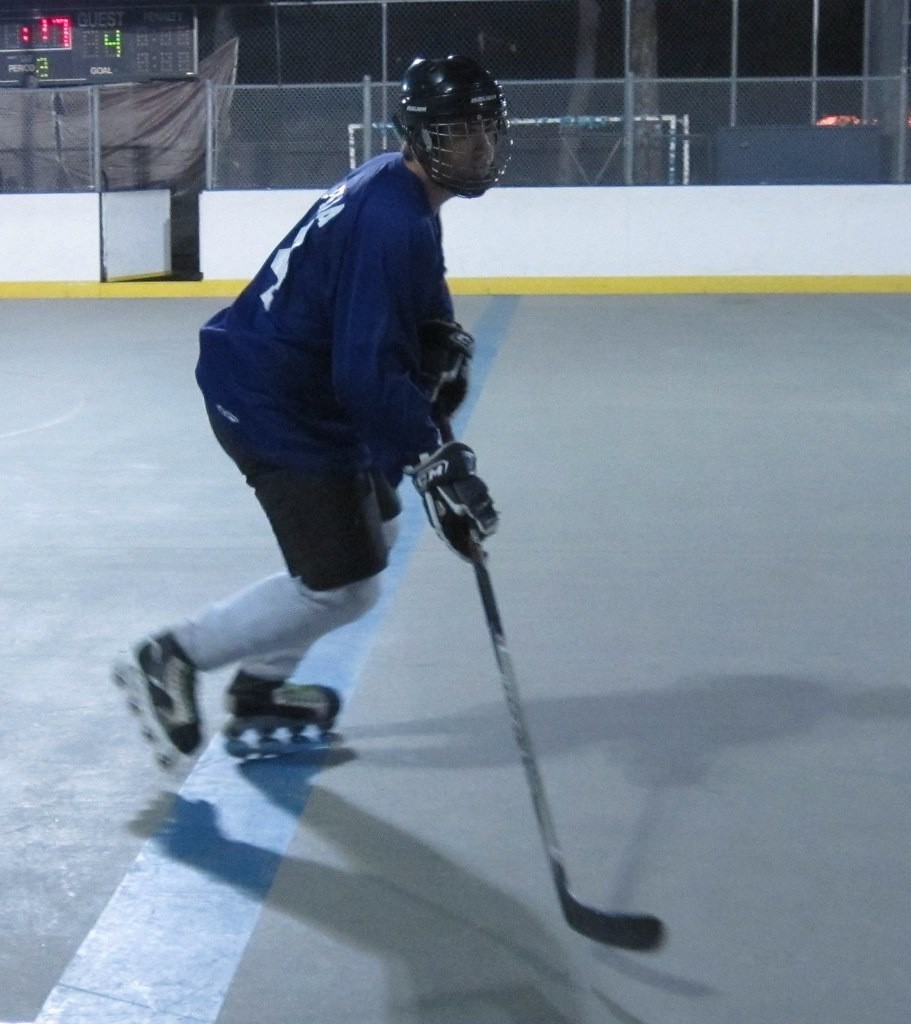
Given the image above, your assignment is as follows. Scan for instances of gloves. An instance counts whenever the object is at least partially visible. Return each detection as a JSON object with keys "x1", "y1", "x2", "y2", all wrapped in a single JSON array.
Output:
[
  {"x1": 415, "y1": 319, "x2": 474, "y2": 418},
  {"x1": 402, "y1": 442, "x2": 498, "y2": 563}
]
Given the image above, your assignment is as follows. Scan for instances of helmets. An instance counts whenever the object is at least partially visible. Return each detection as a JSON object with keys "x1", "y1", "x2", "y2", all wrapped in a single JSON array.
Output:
[{"x1": 393, "y1": 53, "x2": 513, "y2": 200}]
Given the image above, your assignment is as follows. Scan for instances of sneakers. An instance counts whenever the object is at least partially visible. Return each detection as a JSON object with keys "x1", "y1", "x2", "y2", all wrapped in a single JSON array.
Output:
[
  {"x1": 136, "y1": 630, "x2": 203, "y2": 756},
  {"x1": 226, "y1": 670, "x2": 342, "y2": 730}
]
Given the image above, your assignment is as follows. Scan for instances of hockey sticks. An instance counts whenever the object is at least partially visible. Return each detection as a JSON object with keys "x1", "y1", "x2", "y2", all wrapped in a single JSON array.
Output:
[{"x1": 435, "y1": 407, "x2": 669, "y2": 952}]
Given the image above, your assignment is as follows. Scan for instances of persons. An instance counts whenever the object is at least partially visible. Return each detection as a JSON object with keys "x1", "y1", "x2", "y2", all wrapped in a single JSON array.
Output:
[{"x1": 107, "y1": 53, "x2": 514, "y2": 773}]
